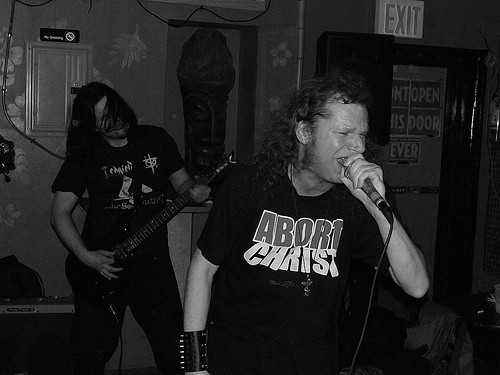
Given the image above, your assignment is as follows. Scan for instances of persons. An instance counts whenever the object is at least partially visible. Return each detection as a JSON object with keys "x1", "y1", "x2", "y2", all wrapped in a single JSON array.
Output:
[
  {"x1": 179, "y1": 64, "x2": 431, "y2": 375},
  {"x1": 50, "y1": 81, "x2": 211, "y2": 375}
]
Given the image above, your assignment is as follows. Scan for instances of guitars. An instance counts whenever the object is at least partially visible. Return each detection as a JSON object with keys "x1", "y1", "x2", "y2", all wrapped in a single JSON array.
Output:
[{"x1": 64, "y1": 150, "x2": 238, "y2": 311}]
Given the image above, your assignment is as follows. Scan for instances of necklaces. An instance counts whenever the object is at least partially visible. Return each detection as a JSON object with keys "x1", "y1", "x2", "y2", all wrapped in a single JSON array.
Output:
[{"x1": 291, "y1": 162, "x2": 335, "y2": 297}]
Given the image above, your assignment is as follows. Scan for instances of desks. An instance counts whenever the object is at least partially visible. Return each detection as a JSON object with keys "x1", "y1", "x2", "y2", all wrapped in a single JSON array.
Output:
[{"x1": 469, "y1": 293, "x2": 500, "y2": 375}]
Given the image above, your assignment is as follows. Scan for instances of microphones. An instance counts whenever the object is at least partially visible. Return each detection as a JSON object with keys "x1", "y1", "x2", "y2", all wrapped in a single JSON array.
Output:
[{"x1": 344, "y1": 161, "x2": 394, "y2": 224}]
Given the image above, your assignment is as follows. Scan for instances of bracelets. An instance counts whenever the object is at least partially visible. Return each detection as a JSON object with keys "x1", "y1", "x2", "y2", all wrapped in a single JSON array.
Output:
[{"x1": 179, "y1": 329, "x2": 209, "y2": 372}]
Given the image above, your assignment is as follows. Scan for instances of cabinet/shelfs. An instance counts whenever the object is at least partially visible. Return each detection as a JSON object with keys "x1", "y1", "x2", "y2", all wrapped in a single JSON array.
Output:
[{"x1": 156, "y1": 202, "x2": 211, "y2": 314}]
